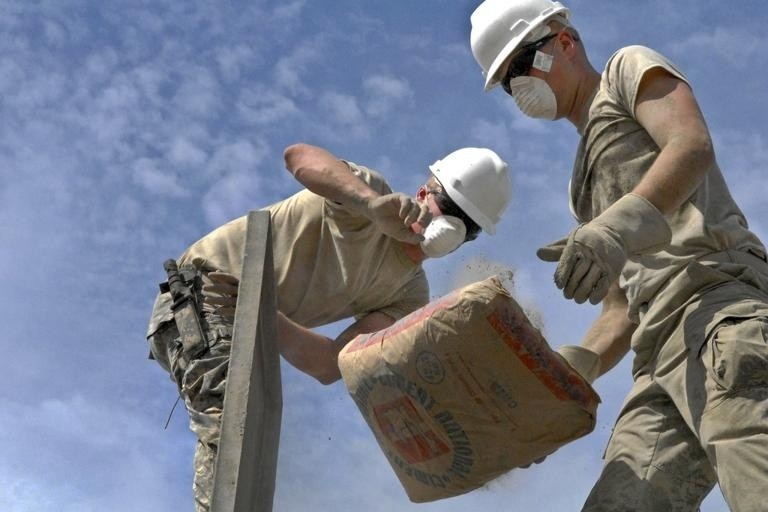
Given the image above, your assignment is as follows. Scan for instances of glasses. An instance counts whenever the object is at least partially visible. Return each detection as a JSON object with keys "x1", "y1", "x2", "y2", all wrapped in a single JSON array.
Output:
[
  {"x1": 499, "y1": 31, "x2": 579, "y2": 98},
  {"x1": 423, "y1": 189, "x2": 483, "y2": 242}
]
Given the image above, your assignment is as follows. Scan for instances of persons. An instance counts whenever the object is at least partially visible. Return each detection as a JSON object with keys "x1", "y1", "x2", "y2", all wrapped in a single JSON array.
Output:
[
  {"x1": 467, "y1": 0, "x2": 768, "y2": 511},
  {"x1": 144, "y1": 142, "x2": 511, "y2": 510}
]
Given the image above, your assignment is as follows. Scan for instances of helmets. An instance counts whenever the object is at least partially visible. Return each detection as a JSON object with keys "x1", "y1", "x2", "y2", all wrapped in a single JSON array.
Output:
[
  {"x1": 426, "y1": 146, "x2": 514, "y2": 237},
  {"x1": 467, "y1": 0, "x2": 572, "y2": 93}
]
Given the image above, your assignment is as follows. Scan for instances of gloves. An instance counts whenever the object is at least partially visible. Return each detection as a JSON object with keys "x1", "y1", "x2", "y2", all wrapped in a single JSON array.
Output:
[
  {"x1": 534, "y1": 192, "x2": 673, "y2": 307},
  {"x1": 516, "y1": 344, "x2": 601, "y2": 470}
]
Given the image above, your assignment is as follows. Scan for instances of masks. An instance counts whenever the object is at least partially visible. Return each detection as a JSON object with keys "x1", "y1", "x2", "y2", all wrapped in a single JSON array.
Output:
[
  {"x1": 418, "y1": 214, "x2": 467, "y2": 259},
  {"x1": 508, "y1": 74, "x2": 557, "y2": 121}
]
